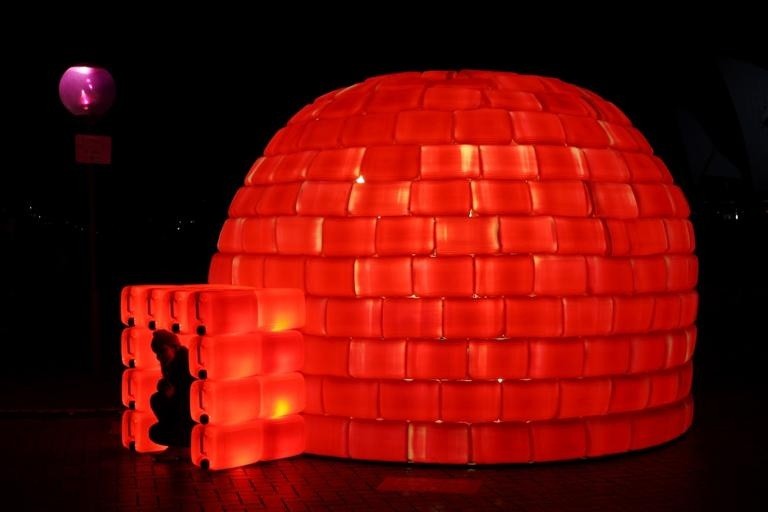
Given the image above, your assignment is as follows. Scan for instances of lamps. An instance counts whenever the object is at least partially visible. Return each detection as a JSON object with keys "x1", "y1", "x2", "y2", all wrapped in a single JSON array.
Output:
[{"x1": 58, "y1": 66, "x2": 116, "y2": 116}]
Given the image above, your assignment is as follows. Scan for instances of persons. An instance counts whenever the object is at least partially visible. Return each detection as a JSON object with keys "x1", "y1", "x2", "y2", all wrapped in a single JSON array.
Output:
[{"x1": 148, "y1": 328, "x2": 197, "y2": 464}]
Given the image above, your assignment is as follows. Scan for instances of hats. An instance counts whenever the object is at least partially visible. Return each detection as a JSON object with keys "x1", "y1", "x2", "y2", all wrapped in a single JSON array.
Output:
[{"x1": 151, "y1": 329, "x2": 179, "y2": 351}]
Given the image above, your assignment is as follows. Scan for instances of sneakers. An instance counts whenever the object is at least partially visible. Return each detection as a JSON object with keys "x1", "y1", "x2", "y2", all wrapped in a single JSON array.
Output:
[{"x1": 156, "y1": 446, "x2": 189, "y2": 463}]
